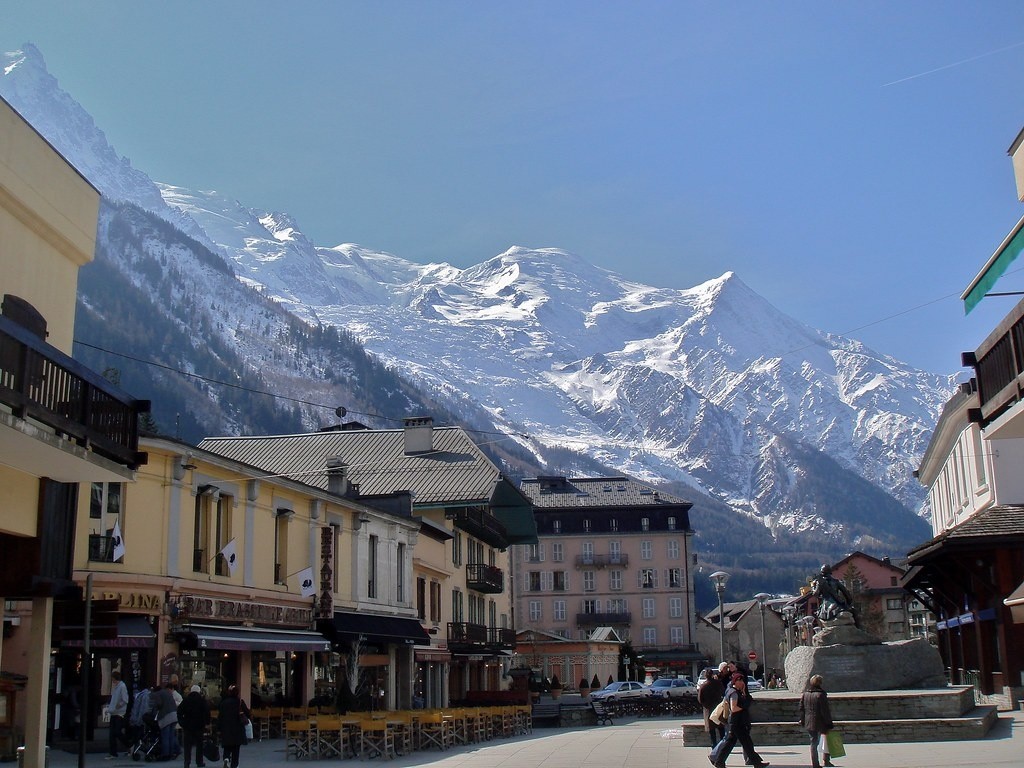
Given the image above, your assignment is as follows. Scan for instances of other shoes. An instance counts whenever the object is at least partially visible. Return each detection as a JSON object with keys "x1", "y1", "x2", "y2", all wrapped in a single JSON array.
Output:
[
  {"x1": 104, "y1": 754, "x2": 117, "y2": 760},
  {"x1": 754, "y1": 762, "x2": 770, "y2": 768},
  {"x1": 714, "y1": 762, "x2": 727, "y2": 768},
  {"x1": 196, "y1": 762, "x2": 206, "y2": 767},
  {"x1": 823, "y1": 761, "x2": 835, "y2": 767},
  {"x1": 223, "y1": 758, "x2": 240, "y2": 768},
  {"x1": 812, "y1": 765, "x2": 822, "y2": 768}
]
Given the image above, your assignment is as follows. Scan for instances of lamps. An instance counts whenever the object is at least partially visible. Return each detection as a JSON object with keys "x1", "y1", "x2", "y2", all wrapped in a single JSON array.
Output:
[
  {"x1": 181, "y1": 464, "x2": 197, "y2": 470},
  {"x1": 359, "y1": 519, "x2": 371, "y2": 523},
  {"x1": 277, "y1": 509, "x2": 296, "y2": 519},
  {"x1": 883, "y1": 555, "x2": 911, "y2": 564},
  {"x1": 198, "y1": 485, "x2": 220, "y2": 497}
]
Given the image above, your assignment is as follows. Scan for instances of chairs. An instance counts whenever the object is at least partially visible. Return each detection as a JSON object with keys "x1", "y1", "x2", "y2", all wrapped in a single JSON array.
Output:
[{"x1": 204, "y1": 705, "x2": 533, "y2": 761}]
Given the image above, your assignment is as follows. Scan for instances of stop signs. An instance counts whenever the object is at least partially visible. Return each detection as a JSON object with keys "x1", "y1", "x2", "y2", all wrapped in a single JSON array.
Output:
[{"x1": 748, "y1": 651, "x2": 757, "y2": 660}]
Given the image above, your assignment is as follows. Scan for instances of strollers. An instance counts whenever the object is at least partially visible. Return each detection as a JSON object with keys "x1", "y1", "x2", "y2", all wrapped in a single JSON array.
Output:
[{"x1": 131, "y1": 709, "x2": 163, "y2": 762}]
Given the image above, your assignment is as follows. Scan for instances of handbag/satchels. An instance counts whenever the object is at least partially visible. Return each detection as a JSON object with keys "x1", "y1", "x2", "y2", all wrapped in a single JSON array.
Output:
[
  {"x1": 245, "y1": 720, "x2": 255, "y2": 739},
  {"x1": 710, "y1": 701, "x2": 729, "y2": 725},
  {"x1": 817, "y1": 731, "x2": 847, "y2": 758},
  {"x1": 202, "y1": 742, "x2": 221, "y2": 762}
]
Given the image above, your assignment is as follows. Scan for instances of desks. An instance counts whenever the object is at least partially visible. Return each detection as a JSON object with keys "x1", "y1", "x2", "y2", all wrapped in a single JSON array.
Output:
[
  {"x1": 309, "y1": 720, "x2": 360, "y2": 756},
  {"x1": 442, "y1": 715, "x2": 454, "y2": 717},
  {"x1": 386, "y1": 721, "x2": 403, "y2": 756},
  {"x1": 372, "y1": 715, "x2": 386, "y2": 719}
]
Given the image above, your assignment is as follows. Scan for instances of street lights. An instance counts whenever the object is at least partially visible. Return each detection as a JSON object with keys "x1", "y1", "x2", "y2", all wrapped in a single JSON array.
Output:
[
  {"x1": 708, "y1": 571, "x2": 732, "y2": 661},
  {"x1": 803, "y1": 615, "x2": 814, "y2": 646},
  {"x1": 753, "y1": 592, "x2": 772, "y2": 689},
  {"x1": 795, "y1": 619, "x2": 805, "y2": 646},
  {"x1": 782, "y1": 606, "x2": 797, "y2": 651}
]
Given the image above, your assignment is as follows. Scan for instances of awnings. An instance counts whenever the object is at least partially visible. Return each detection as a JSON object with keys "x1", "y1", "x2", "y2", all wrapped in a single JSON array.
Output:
[
  {"x1": 58, "y1": 616, "x2": 156, "y2": 648},
  {"x1": 183, "y1": 623, "x2": 331, "y2": 652},
  {"x1": 332, "y1": 611, "x2": 431, "y2": 647}
]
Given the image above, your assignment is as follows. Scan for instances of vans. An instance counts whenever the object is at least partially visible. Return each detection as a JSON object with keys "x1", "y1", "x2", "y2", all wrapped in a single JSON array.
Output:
[{"x1": 696, "y1": 668, "x2": 719, "y2": 690}]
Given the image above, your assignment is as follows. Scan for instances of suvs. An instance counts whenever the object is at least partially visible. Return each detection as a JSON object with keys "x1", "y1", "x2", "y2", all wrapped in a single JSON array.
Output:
[{"x1": 649, "y1": 679, "x2": 694, "y2": 697}]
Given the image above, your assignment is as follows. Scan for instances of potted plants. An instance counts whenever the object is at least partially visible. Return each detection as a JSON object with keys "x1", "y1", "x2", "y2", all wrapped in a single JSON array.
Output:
[
  {"x1": 591, "y1": 673, "x2": 600, "y2": 691},
  {"x1": 579, "y1": 679, "x2": 589, "y2": 698},
  {"x1": 531, "y1": 679, "x2": 539, "y2": 697},
  {"x1": 550, "y1": 675, "x2": 562, "y2": 696}
]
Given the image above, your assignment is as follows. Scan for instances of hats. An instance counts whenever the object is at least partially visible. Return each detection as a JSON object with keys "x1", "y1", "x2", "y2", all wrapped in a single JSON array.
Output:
[
  {"x1": 731, "y1": 673, "x2": 743, "y2": 684},
  {"x1": 191, "y1": 685, "x2": 201, "y2": 692},
  {"x1": 719, "y1": 662, "x2": 728, "y2": 672}
]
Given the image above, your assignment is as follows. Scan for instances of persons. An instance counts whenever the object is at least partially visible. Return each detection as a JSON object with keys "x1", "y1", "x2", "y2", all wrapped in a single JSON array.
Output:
[
  {"x1": 698, "y1": 661, "x2": 782, "y2": 765},
  {"x1": 799, "y1": 675, "x2": 834, "y2": 768},
  {"x1": 713, "y1": 673, "x2": 770, "y2": 768},
  {"x1": 308, "y1": 687, "x2": 328, "y2": 715},
  {"x1": 103, "y1": 671, "x2": 250, "y2": 768},
  {"x1": 812, "y1": 564, "x2": 852, "y2": 619}
]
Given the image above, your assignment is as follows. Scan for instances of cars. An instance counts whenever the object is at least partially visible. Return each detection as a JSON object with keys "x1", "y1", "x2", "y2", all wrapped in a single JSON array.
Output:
[
  {"x1": 590, "y1": 681, "x2": 652, "y2": 703},
  {"x1": 747, "y1": 676, "x2": 762, "y2": 693},
  {"x1": 691, "y1": 683, "x2": 699, "y2": 696}
]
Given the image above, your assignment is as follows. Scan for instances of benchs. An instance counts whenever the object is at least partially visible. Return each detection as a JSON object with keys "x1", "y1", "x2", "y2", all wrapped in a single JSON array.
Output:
[
  {"x1": 591, "y1": 702, "x2": 615, "y2": 725},
  {"x1": 532, "y1": 705, "x2": 561, "y2": 727}
]
